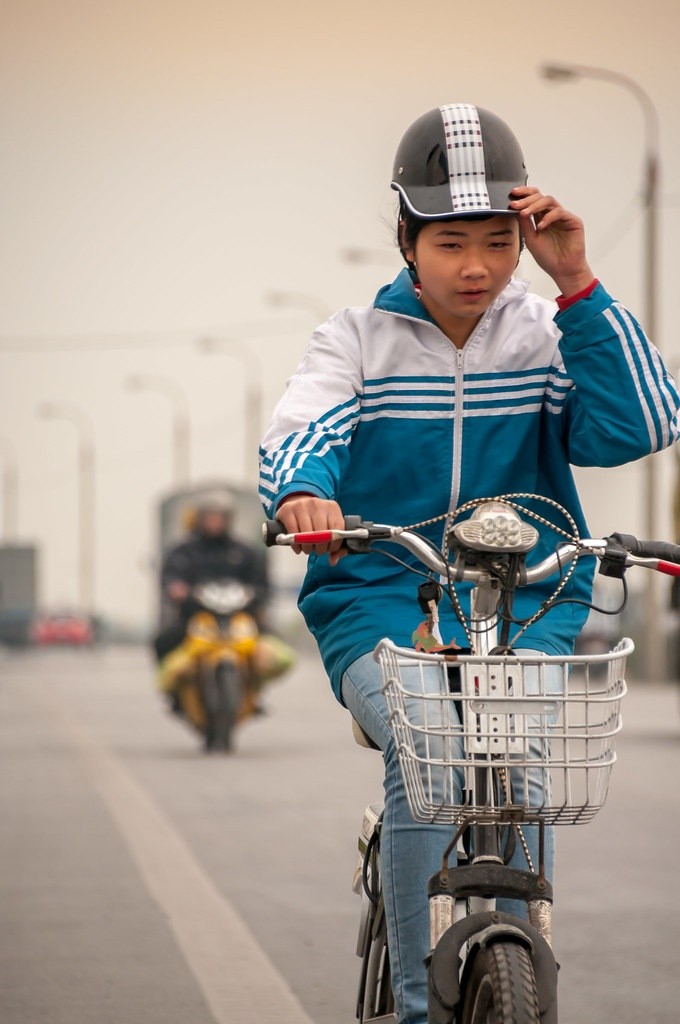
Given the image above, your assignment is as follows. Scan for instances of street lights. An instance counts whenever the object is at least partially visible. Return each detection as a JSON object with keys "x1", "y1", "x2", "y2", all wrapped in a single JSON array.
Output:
[{"x1": 542, "y1": 57, "x2": 663, "y2": 683}]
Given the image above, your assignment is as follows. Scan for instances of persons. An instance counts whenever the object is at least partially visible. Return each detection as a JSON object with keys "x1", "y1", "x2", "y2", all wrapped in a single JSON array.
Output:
[
  {"x1": 152, "y1": 494, "x2": 274, "y2": 717},
  {"x1": 258, "y1": 104, "x2": 680, "y2": 1024}
]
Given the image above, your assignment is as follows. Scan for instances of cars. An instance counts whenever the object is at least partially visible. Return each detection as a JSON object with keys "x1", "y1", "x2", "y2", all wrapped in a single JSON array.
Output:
[{"x1": 28, "y1": 606, "x2": 97, "y2": 648}]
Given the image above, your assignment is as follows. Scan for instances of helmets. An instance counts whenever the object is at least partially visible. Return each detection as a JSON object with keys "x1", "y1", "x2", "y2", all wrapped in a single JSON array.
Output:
[
  {"x1": 192, "y1": 487, "x2": 233, "y2": 537},
  {"x1": 390, "y1": 108, "x2": 528, "y2": 217}
]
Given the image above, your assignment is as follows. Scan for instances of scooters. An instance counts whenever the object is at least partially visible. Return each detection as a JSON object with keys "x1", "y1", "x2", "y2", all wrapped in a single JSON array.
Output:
[
  {"x1": 260, "y1": 514, "x2": 680, "y2": 1023},
  {"x1": 170, "y1": 574, "x2": 272, "y2": 755}
]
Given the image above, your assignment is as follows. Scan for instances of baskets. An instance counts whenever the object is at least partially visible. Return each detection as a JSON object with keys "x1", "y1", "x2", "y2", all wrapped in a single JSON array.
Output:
[{"x1": 375, "y1": 635, "x2": 635, "y2": 826}]
